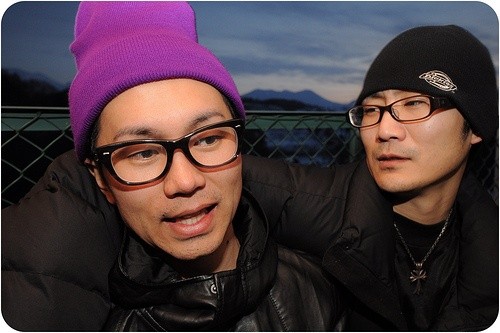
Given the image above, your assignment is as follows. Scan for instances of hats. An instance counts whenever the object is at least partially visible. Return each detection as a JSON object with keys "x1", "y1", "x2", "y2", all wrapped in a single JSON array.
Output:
[
  {"x1": 353, "y1": 24, "x2": 499, "y2": 142},
  {"x1": 68, "y1": 1, "x2": 247, "y2": 161}
]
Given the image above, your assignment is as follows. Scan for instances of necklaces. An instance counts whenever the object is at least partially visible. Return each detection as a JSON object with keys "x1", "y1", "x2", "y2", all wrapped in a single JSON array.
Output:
[{"x1": 392, "y1": 208, "x2": 452, "y2": 292}]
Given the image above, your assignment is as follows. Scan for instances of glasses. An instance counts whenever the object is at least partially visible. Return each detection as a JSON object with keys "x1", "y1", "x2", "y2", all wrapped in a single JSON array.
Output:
[
  {"x1": 346, "y1": 94, "x2": 456, "y2": 129},
  {"x1": 89, "y1": 117, "x2": 244, "y2": 187}
]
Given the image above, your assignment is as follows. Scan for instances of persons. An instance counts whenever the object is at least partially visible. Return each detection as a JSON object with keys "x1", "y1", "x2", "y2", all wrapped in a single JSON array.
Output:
[
  {"x1": 67, "y1": 1, "x2": 350, "y2": 332},
  {"x1": 1, "y1": 25, "x2": 499, "y2": 332}
]
